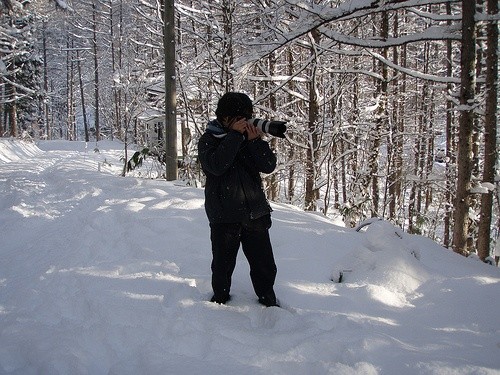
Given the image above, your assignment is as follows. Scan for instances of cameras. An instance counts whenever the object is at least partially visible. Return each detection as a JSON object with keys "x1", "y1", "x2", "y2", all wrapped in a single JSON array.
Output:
[{"x1": 237, "y1": 113, "x2": 287, "y2": 138}]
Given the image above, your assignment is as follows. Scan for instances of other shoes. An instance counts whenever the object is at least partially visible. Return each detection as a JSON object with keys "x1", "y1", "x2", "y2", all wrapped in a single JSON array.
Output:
[{"x1": 210, "y1": 293, "x2": 230, "y2": 303}]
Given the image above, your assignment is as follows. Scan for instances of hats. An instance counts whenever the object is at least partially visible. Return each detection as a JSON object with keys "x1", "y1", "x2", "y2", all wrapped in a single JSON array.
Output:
[{"x1": 215, "y1": 92, "x2": 253, "y2": 118}]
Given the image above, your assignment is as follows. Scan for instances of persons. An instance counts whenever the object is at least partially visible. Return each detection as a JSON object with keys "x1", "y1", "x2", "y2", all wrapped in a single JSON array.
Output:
[{"x1": 199, "y1": 93, "x2": 280, "y2": 307}]
